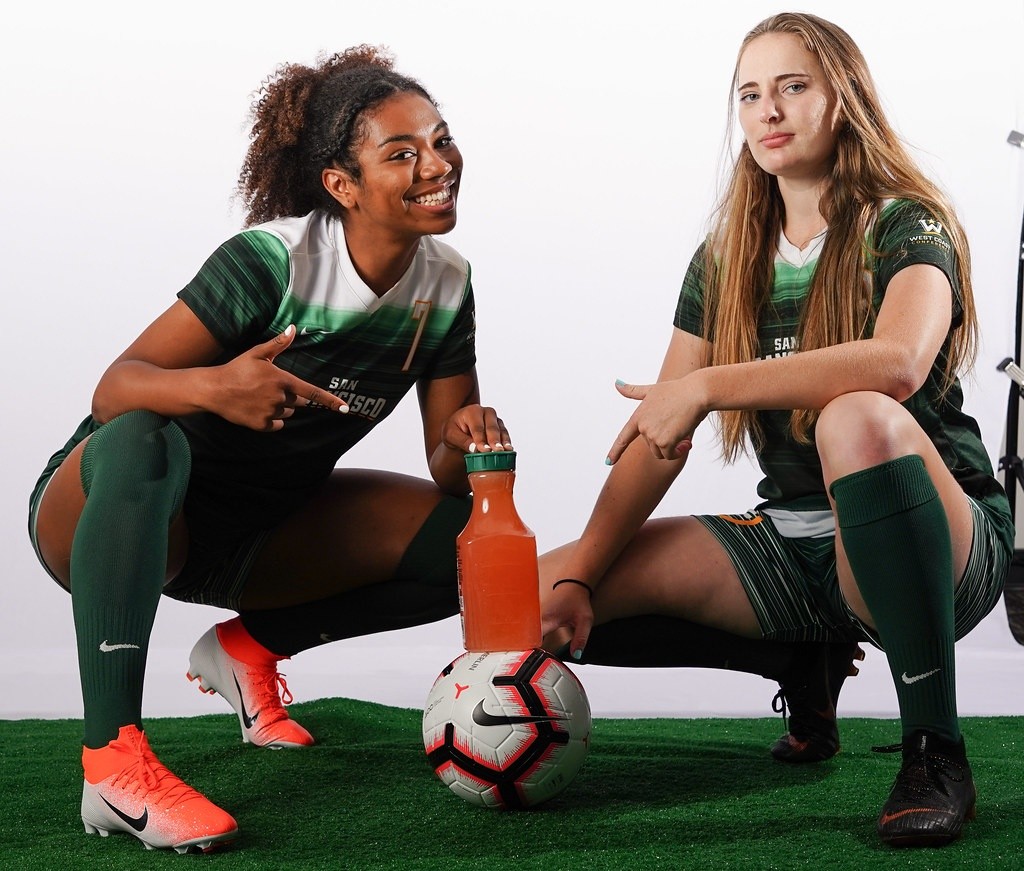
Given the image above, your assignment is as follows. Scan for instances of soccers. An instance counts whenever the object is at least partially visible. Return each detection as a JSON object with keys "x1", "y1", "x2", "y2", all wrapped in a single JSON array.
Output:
[{"x1": 422, "y1": 648, "x2": 591, "y2": 810}]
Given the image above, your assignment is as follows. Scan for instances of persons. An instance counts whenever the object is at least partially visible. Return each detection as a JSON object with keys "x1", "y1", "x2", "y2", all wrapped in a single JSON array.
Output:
[
  {"x1": 27, "y1": 43, "x2": 516, "y2": 857},
  {"x1": 537, "y1": 12, "x2": 1014, "y2": 851}
]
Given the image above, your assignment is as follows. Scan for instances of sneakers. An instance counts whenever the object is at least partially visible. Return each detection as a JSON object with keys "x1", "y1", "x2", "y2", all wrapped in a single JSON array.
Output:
[
  {"x1": 877, "y1": 731, "x2": 976, "y2": 846},
  {"x1": 81, "y1": 723, "x2": 239, "y2": 855},
  {"x1": 769, "y1": 642, "x2": 865, "y2": 762},
  {"x1": 187, "y1": 618, "x2": 317, "y2": 751}
]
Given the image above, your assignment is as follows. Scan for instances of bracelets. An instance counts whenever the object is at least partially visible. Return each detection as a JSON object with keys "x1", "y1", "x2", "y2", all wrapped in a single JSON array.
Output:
[{"x1": 552, "y1": 579, "x2": 592, "y2": 600}]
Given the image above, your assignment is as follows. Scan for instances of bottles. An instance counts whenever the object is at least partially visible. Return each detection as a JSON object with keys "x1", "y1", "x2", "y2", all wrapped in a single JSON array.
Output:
[{"x1": 456, "y1": 448, "x2": 545, "y2": 652}]
